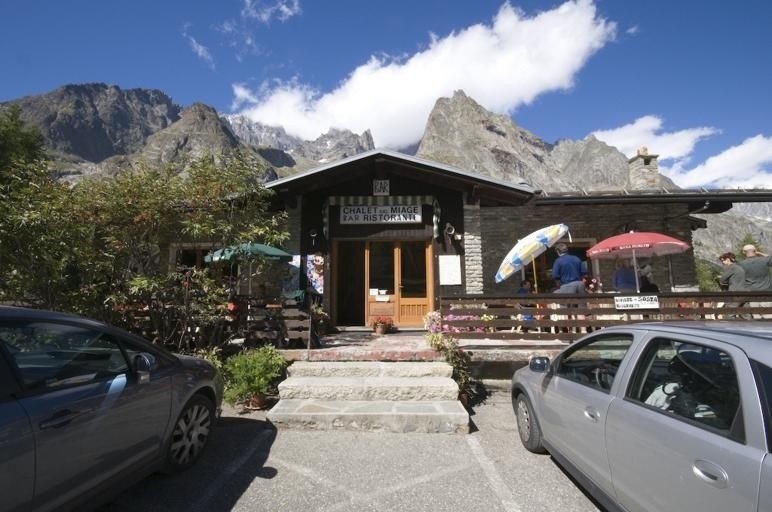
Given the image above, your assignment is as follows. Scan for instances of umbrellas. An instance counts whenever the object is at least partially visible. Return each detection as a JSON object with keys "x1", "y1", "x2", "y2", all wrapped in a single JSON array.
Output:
[
  {"x1": 586, "y1": 230, "x2": 690, "y2": 320},
  {"x1": 204, "y1": 241, "x2": 293, "y2": 320},
  {"x1": 495, "y1": 222, "x2": 570, "y2": 332}
]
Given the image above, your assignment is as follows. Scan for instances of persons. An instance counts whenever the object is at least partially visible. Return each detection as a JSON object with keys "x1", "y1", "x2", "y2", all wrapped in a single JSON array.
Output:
[
  {"x1": 640, "y1": 276, "x2": 658, "y2": 292},
  {"x1": 552, "y1": 242, "x2": 587, "y2": 333},
  {"x1": 612, "y1": 258, "x2": 636, "y2": 293},
  {"x1": 719, "y1": 252, "x2": 753, "y2": 319},
  {"x1": 740, "y1": 244, "x2": 772, "y2": 319},
  {"x1": 517, "y1": 280, "x2": 532, "y2": 294}
]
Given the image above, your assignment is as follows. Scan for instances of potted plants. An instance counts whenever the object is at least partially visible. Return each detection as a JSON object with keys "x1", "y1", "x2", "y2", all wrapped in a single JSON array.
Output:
[
  {"x1": 374, "y1": 316, "x2": 387, "y2": 335},
  {"x1": 427, "y1": 332, "x2": 479, "y2": 406},
  {"x1": 220, "y1": 343, "x2": 289, "y2": 410}
]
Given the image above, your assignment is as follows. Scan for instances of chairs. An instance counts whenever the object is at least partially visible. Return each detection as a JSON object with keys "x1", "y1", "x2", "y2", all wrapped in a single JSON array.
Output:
[
  {"x1": 510, "y1": 303, "x2": 542, "y2": 333},
  {"x1": 547, "y1": 290, "x2": 625, "y2": 333},
  {"x1": 644, "y1": 351, "x2": 715, "y2": 415}
]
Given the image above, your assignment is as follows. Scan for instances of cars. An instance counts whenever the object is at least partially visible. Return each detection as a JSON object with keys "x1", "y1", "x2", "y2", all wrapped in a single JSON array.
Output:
[
  {"x1": 511, "y1": 321, "x2": 772, "y2": 512},
  {"x1": 0, "y1": 305, "x2": 224, "y2": 511}
]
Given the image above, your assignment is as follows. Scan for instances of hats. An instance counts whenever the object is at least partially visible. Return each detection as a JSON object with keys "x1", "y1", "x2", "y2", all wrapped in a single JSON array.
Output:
[{"x1": 743, "y1": 245, "x2": 755, "y2": 253}]
